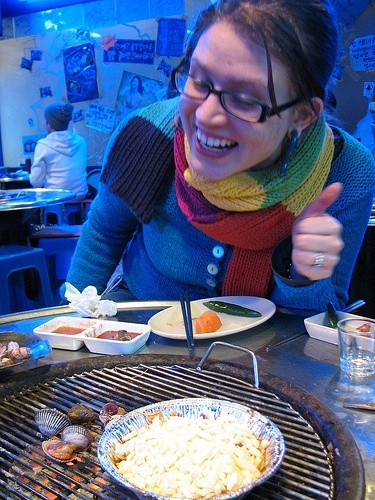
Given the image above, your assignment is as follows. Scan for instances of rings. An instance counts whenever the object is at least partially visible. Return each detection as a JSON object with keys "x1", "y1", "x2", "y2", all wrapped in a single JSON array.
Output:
[{"x1": 315, "y1": 255, "x2": 324, "y2": 265}]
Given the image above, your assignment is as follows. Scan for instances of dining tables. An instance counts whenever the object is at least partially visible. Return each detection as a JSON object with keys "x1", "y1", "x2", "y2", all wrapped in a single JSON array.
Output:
[{"x1": 0, "y1": 301, "x2": 375, "y2": 500}]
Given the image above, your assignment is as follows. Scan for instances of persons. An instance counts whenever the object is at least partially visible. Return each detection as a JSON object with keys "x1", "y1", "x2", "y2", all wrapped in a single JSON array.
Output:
[
  {"x1": 118, "y1": 77, "x2": 144, "y2": 110},
  {"x1": 29, "y1": 104, "x2": 88, "y2": 200},
  {"x1": 59, "y1": 0, "x2": 375, "y2": 313}
]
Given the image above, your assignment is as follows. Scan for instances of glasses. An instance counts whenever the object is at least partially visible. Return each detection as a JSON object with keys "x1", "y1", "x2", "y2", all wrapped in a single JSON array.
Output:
[{"x1": 171, "y1": 60, "x2": 300, "y2": 123}]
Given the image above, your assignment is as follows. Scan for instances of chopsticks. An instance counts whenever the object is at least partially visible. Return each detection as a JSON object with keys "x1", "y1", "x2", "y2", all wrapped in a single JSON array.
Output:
[{"x1": 180, "y1": 291, "x2": 194, "y2": 351}]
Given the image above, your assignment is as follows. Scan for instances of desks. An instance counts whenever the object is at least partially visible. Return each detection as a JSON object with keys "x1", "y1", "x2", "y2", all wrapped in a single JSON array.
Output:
[{"x1": 0, "y1": 173, "x2": 76, "y2": 244}]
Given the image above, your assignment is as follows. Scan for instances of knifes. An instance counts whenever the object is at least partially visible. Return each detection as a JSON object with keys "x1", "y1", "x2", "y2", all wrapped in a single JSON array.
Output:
[{"x1": 264, "y1": 300, "x2": 365, "y2": 350}]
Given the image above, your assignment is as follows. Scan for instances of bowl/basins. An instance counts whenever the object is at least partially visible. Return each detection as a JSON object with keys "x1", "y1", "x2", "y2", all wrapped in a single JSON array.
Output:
[
  {"x1": 96, "y1": 398, "x2": 286, "y2": 500},
  {"x1": 0, "y1": 331, "x2": 41, "y2": 370}
]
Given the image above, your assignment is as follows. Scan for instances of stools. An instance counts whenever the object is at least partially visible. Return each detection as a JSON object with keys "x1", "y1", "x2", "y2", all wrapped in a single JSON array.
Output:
[
  {"x1": 43, "y1": 205, "x2": 84, "y2": 227},
  {"x1": 0, "y1": 243, "x2": 57, "y2": 315},
  {"x1": 39, "y1": 237, "x2": 81, "y2": 306}
]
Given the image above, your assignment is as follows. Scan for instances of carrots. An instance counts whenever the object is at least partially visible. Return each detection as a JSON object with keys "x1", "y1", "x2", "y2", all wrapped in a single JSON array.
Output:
[{"x1": 195, "y1": 310, "x2": 222, "y2": 333}]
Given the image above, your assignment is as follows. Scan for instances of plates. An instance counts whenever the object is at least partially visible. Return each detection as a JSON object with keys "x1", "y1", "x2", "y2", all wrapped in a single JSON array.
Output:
[
  {"x1": 147, "y1": 296, "x2": 276, "y2": 340},
  {"x1": 33, "y1": 316, "x2": 152, "y2": 355},
  {"x1": 304, "y1": 310, "x2": 375, "y2": 345}
]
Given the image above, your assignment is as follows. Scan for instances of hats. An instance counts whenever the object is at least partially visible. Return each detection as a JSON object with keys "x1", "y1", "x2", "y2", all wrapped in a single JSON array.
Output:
[{"x1": 44, "y1": 102, "x2": 74, "y2": 130}]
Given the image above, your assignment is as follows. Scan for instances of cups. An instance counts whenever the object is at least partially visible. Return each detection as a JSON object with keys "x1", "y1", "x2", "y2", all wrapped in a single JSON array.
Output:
[
  {"x1": 337, "y1": 318, "x2": 375, "y2": 377},
  {"x1": 26, "y1": 157, "x2": 31, "y2": 173}
]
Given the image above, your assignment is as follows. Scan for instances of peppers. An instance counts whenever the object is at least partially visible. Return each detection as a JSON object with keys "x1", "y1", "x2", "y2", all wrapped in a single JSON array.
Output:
[
  {"x1": 202, "y1": 301, "x2": 263, "y2": 317},
  {"x1": 327, "y1": 301, "x2": 341, "y2": 329}
]
